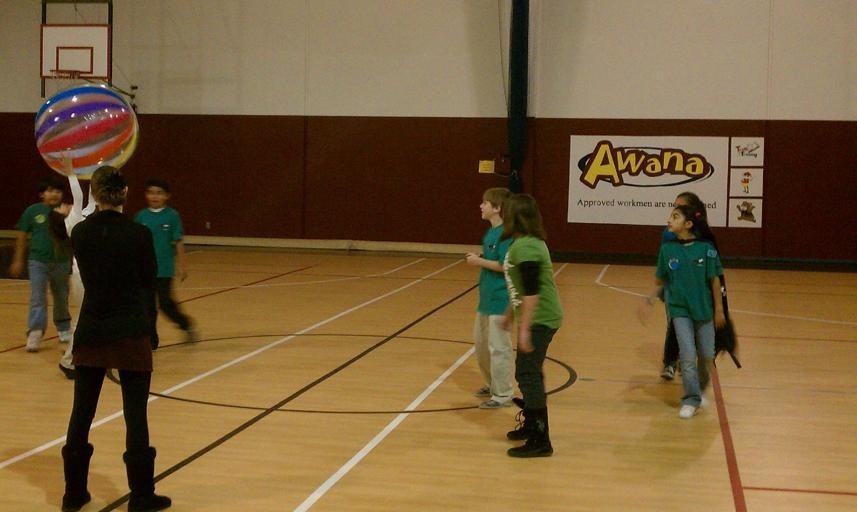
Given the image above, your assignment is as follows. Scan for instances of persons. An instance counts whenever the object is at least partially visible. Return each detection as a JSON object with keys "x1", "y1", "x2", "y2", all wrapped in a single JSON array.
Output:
[
  {"x1": 658, "y1": 192, "x2": 725, "y2": 378},
  {"x1": 61, "y1": 166, "x2": 172, "y2": 511},
  {"x1": 653, "y1": 205, "x2": 727, "y2": 419},
  {"x1": 47, "y1": 172, "x2": 97, "y2": 380},
  {"x1": 7, "y1": 174, "x2": 72, "y2": 352},
  {"x1": 495, "y1": 193, "x2": 563, "y2": 458},
  {"x1": 463, "y1": 186, "x2": 514, "y2": 409},
  {"x1": 132, "y1": 175, "x2": 197, "y2": 344}
]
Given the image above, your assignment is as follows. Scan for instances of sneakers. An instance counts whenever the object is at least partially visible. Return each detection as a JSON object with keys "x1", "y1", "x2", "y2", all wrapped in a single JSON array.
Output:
[
  {"x1": 26, "y1": 330, "x2": 42, "y2": 352},
  {"x1": 475, "y1": 386, "x2": 491, "y2": 397},
  {"x1": 58, "y1": 331, "x2": 71, "y2": 342},
  {"x1": 480, "y1": 398, "x2": 502, "y2": 408},
  {"x1": 661, "y1": 361, "x2": 677, "y2": 379},
  {"x1": 679, "y1": 404, "x2": 696, "y2": 417}
]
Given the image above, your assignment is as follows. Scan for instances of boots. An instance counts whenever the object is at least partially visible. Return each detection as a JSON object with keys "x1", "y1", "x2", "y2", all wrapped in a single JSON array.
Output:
[
  {"x1": 62, "y1": 443, "x2": 94, "y2": 512},
  {"x1": 507, "y1": 398, "x2": 553, "y2": 456},
  {"x1": 122, "y1": 447, "x2": 172, "y2": 511}
]
